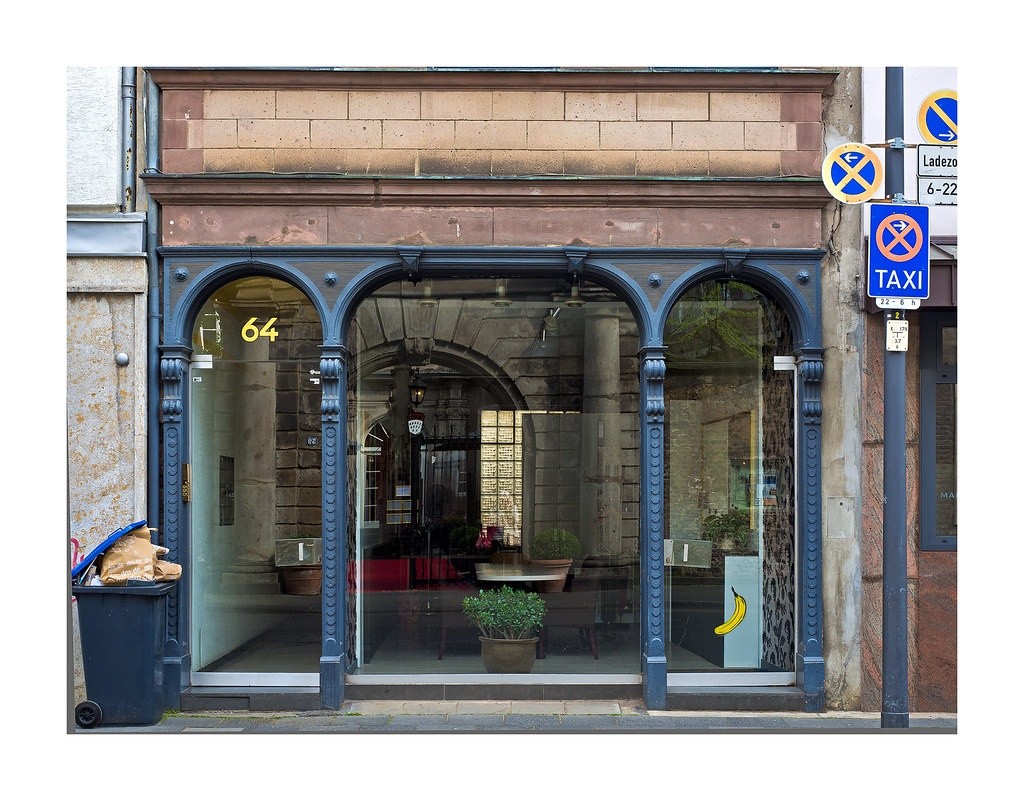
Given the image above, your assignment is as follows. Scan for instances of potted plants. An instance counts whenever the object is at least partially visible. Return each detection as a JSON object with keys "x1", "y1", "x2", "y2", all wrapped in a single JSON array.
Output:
[
  {"x1": 530, "y1": 528, "x2": 579, "y2": 592},
  {"x1": 463, "y1": 585, "x2": 549, "y2": 674}
]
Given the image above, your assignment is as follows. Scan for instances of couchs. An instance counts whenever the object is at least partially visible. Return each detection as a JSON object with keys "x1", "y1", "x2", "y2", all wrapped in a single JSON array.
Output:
[{"x1": 537, "y1": 555, "x2": 609, "y2": 659}]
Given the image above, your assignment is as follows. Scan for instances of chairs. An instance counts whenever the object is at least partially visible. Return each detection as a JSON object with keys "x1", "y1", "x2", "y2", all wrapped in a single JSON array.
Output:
[{"x1": 438, "y1": 592, "x2": 470, "y2": 659}]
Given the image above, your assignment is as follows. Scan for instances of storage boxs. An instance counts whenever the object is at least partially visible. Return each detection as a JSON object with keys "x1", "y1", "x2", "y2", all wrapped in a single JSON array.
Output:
[{"x1": 274, "y1": 538, "x2": 321, "y2": 566}]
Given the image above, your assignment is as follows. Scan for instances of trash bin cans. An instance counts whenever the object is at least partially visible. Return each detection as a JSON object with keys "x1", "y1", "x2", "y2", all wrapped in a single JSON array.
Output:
[{"x1": 72, "y1": 520, "x2": 178, "y2": 729}]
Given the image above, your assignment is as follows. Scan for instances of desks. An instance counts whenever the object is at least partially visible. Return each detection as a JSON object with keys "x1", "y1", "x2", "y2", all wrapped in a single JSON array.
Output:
[{"x1": 476, "y1": 562, "x2": 565, "y2": 592}]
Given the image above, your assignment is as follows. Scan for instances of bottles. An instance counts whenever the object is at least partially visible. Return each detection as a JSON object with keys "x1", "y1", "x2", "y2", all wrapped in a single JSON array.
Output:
[{"x1": 756, "y1": 462, "x2": 777, "y2": 505}]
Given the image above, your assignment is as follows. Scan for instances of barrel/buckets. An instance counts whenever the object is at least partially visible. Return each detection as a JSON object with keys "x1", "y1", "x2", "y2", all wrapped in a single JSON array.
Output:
[{"x1": 487, "y1": 526, "x2": 504, "y2": 544}]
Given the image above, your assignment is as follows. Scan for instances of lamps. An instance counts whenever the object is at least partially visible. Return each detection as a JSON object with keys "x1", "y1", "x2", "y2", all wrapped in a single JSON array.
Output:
[
  {"x1": 409, "y1": 367, "x2": 428, "y2": 408},
  {"x1": 407, "y1": 412, "x2": 425, "y2": 437}
]
